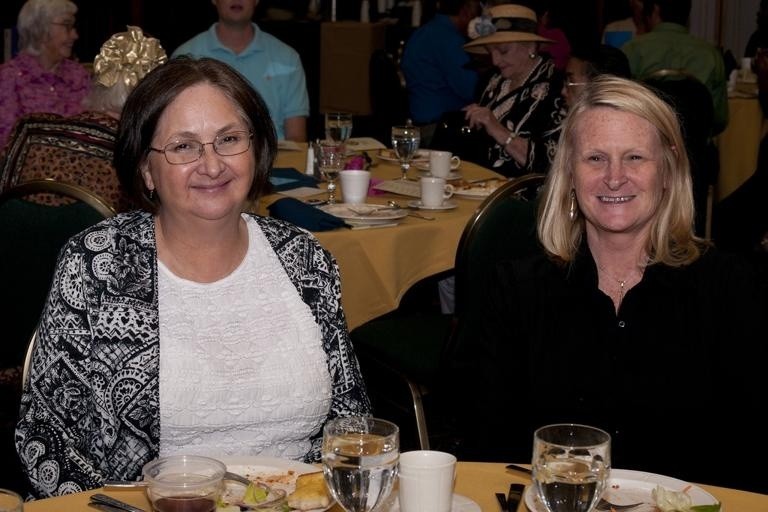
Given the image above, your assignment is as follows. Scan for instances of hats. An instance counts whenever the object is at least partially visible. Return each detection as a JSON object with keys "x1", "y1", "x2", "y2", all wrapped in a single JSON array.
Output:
[{"x1": 461, "y1": 3, "x2": 555, "y2": 54}]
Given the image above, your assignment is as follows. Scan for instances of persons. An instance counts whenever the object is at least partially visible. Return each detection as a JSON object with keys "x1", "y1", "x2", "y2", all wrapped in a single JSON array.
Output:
[
  {"x1": 433, "y1": 74, "x2": 767, "y2": 491},
  {"x1": 14, "y1": 56, "x2": 376, "y2": 498}
]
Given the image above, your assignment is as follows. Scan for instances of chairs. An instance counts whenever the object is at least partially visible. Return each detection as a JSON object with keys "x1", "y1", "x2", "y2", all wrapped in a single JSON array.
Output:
[
  {"x1": 370, "y1": 35, "x2": 439, "y2": 144},
  {"x1": 639, "y1": 69, "x2": 718, "y2": 242},
  {"x1": 2, "y1": 180, "x2": 117, "y2": 383},
  {"x1": 452, "y1": 174, "x2": 551, "y2": 312}
]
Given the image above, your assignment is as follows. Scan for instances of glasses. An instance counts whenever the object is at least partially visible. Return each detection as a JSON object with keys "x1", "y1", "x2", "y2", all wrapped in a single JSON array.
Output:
[{"x1": 150, "y1": 130, "x2": 255, "y2": 166}]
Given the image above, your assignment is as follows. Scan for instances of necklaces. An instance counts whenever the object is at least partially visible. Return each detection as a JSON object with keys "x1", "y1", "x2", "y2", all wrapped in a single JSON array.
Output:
[{"x1": 591, "y1": 257, "x2": 637, "y2": 304}]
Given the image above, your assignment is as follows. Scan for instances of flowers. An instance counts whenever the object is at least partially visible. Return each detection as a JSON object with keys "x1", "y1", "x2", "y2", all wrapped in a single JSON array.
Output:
[{"x1": 466, "y1": 15, "x2": 496, "y2": 40}]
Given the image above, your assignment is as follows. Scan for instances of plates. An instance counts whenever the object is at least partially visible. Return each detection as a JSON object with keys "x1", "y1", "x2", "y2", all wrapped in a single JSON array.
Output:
[
  {"x1": 277, "y1": 135, "x2": 515, "y2": 224},
  {"x1": 524, "y1": 468, "x2": 722, "y2": 511},
  {"x1": 148, "y1": 454, "x2": 340, "y2": 512},
  {"x1": 385, "y1": 491, "x2": 482, "y2": 511}
]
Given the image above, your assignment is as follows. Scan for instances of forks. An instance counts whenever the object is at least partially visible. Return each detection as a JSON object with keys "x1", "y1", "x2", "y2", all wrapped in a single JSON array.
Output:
[
  {"x1": 103, "y1": 470, "x2": 252, "y2": 489},
  {"x1": 503, "y1": 463, "x2": 643, "y2": 511}
]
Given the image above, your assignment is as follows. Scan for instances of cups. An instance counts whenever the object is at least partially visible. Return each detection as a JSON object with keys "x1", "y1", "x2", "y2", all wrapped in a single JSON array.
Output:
[
  {"x1": 532, "y1": 423, "x2": 614, "y2": 511},
  {"x1": 141, "y1": 456, "x2": 225, "y2": 512},
  {"x1": 322, "y1": 417, "x2": 401, "y2": 512},
  {"x1": 399, "y1": 450, "x2": 457, "y2": 511},
  {"x1": 0, "y1": 488, "x2": 24, "y2": 512}
]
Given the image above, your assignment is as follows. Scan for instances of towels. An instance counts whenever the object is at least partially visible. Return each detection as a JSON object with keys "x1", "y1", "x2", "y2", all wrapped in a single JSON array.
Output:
[
  {"x1": 258, "y1": 164, "x2": 322, "y2": 196},
  {"x1": 263, "y1": 197, "x2": 344, "y2": 234}
]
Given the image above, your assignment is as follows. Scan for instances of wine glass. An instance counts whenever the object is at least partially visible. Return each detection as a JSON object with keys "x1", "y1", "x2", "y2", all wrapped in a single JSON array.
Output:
[{"x1": 314, "y1": 109, "x2": 421, "y2": 206}]
